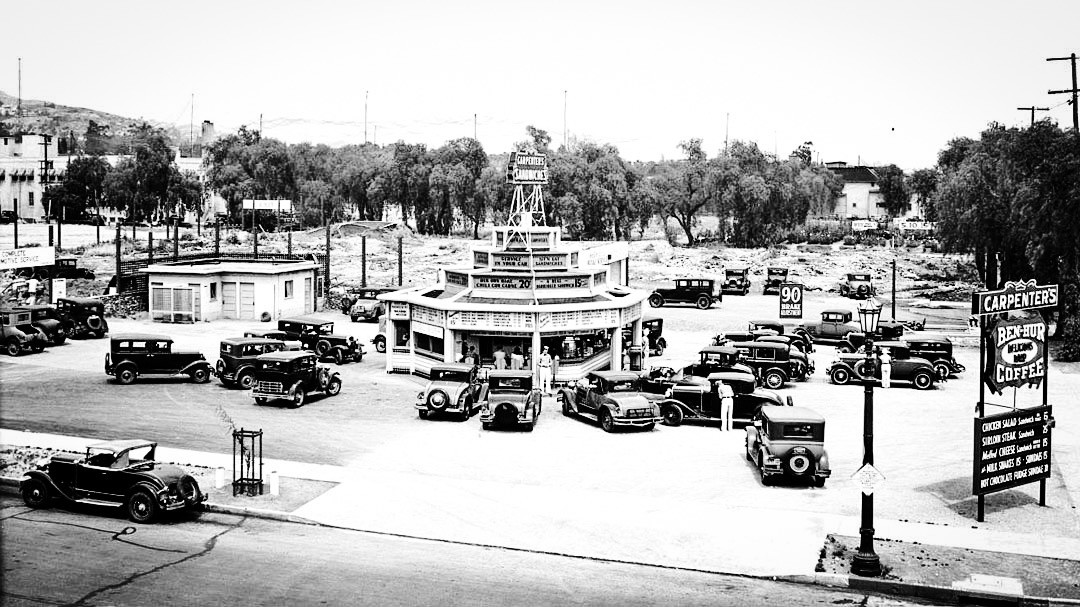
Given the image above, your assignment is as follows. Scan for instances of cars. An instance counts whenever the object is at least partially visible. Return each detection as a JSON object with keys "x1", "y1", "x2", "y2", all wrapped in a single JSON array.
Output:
[
  {"x1": 0, "y1": 211, "x2": 18, "y2": 225},
  {"x1": 248, "y1": 350, "x2": 342, "y2": 408},
  {"x1": 557, "y1": 371, "x2": 664, "y2": 432},
  {"x1": 839, "y1": 273, "x2": 877, "y2": 300},
  {"x1": 622, "y1": 318, "x2": 666, "y2": 356},
  {"x1": 0, "y1": 299, "x2": 109, "y2": 357},
  {"x1": 214, "y1": 318, "x2": 367, "y2": 389},
  {"x1": 791, "y1": 310, "x2": 863, "y2": 345},
  {"x1": 719, "y1": 267, "x2": 750, "y2": 295},
  {"x1": 745, "y1": 395, "x2": 832, "y2": 487},
  {"x1": 349, "y1": 288, "x2": 396, "y2": 322},
  {"x1": 105, "y1": 336, "x2": 215, "y2": 385},
  {"x1": 479, "y1": 368, "x2": 542, "y2": 431},
  {"x1": 20, "y1": 443, "x2": 209, "y2": 523},
  {"x1": 826, "y1": 318, "x2": 965, "y2": 390},
  {"x1": 711, "y1": 321, "x2": 816, "y2": 389},
  {"x1": 647, "y1": 279, "x2": 722, "y2": 310},
  {"x1": 415, "y1": 363, "x2": 497, "y2": 420},
  {"x1": 763, "y1": 267, "x2": 788, "y2": 294},
  {"x1": 33, "y1": 258, "x2": 96, "y2": 281},
  {"x1": 639, "y1": 347, "x2": 794, "y2": 426}
]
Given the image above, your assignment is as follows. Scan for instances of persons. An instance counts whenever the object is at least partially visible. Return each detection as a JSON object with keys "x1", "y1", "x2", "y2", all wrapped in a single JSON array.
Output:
[
  {"x1": 879, "y1": 348, "x2": 892, "y2": 389},
  {"x1": 537, "y1": 348, "x2": 553, "y2": 395},
  {"x1": 717, "y1": 381, "x2": 735, "y2": 432}
]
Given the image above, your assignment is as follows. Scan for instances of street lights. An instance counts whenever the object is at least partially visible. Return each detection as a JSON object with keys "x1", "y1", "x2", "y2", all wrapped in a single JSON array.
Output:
[{"x1": 856, "y1": 292, "x2": 883, "y2": 577}]
